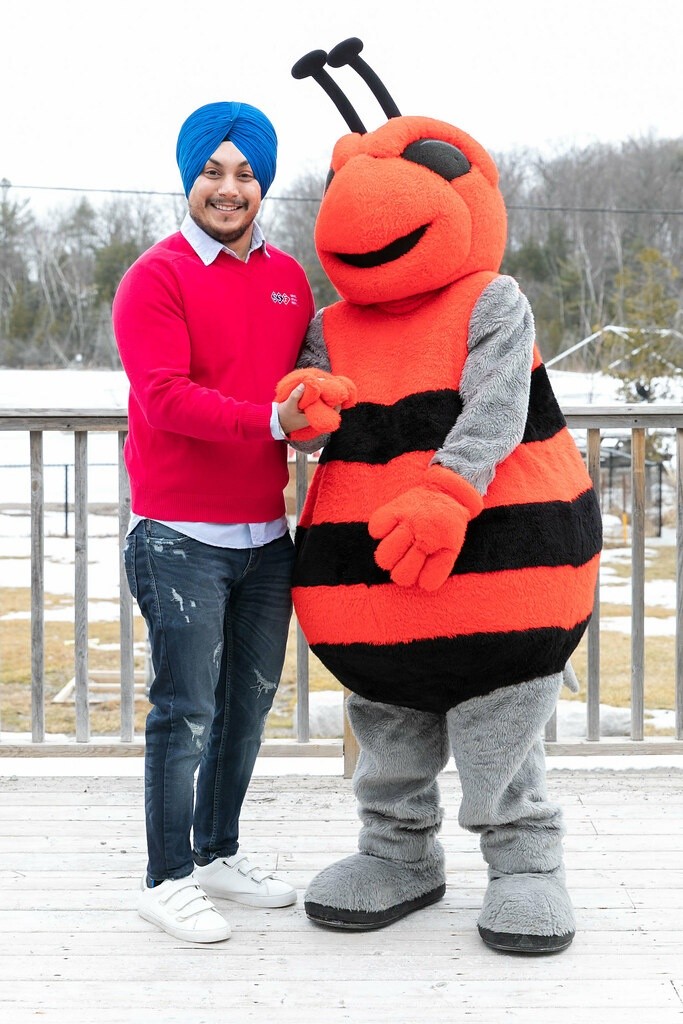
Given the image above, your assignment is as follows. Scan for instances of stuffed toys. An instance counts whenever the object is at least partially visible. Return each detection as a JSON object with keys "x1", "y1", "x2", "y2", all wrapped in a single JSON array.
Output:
[{"x1": 272, "y1": 33, "x2": 603, "y2": 957}]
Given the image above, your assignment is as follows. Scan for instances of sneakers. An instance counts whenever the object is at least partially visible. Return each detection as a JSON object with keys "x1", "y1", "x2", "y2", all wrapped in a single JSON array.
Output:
[{"x1": 139, "y1": 855, "x2": 299, "y2": 946}]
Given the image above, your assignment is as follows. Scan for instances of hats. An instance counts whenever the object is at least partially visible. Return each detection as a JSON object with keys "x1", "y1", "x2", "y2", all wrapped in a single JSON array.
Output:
[{"x1": 176, "y1": 102, "x2": 277, "y2": 198}]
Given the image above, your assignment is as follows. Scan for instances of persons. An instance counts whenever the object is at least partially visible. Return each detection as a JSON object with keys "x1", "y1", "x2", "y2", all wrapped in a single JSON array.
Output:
[{"x1": 115, "y1": 101, "x2": 332, "y2": 946}]
